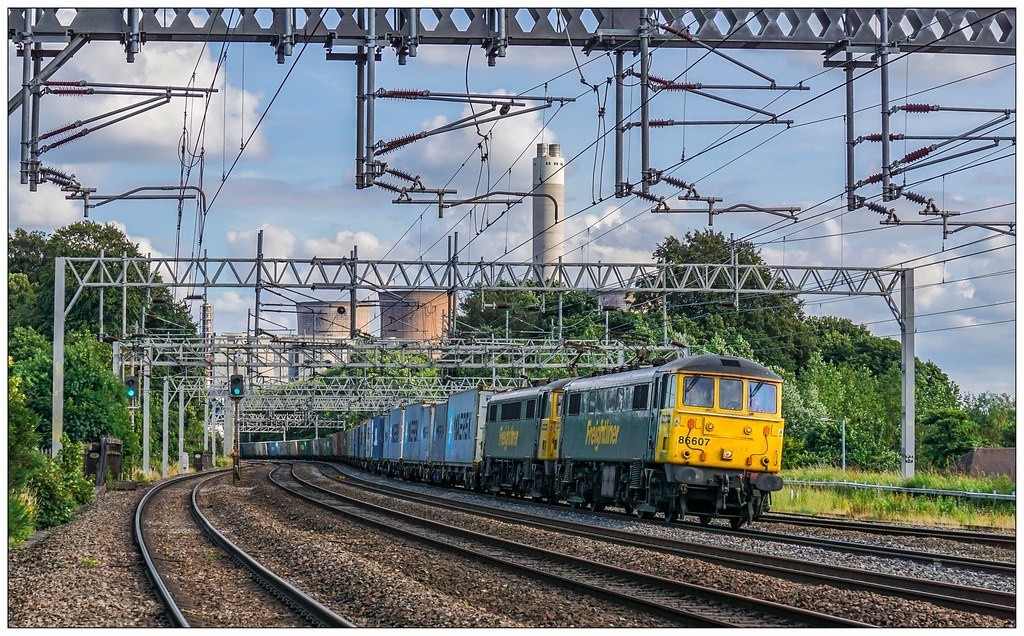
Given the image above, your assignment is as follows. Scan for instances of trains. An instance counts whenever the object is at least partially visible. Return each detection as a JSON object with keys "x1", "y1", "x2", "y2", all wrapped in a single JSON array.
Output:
[{"x1": 238, "y1": 353, "x2": 789, "y2": 531}]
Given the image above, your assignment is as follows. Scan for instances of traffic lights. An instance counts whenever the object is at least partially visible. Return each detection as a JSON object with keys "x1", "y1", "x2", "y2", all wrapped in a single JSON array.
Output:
[
  {"x1": 125, "y1": 375, "x2": 138, "y2": 398},
  {"x1": 230, "y1": 374, "x2": 244, "y2": 397}
]
